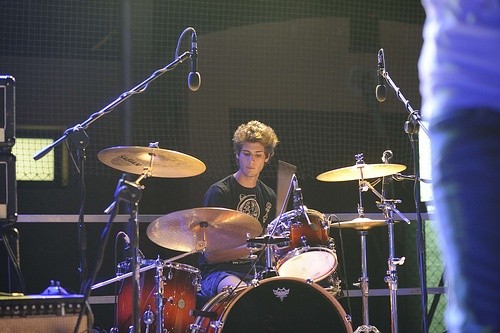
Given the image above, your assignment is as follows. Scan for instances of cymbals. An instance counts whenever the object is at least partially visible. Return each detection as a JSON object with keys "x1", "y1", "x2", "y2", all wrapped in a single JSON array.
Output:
[
  {"x1": 315, "y1": 164, "x2": 407, "y2": 182},
  {"x1": 146, "y1": 207, "x2": 264, "y2": 266},
  {"x1": 97, "y1": 145, "x2": 207, "y2": 179},
  {"x1": 330, "y1": 218, "x2": 401, "y2": 230}
]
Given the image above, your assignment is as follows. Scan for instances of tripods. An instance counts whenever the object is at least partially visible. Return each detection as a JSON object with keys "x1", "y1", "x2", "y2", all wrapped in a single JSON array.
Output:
[{"x1": 353, "y1": 230, "x2": 381, "y2": 333}]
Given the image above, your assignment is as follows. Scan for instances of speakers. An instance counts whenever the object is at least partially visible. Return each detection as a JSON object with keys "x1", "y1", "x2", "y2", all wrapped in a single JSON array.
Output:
[{"x1": 0, "y1": 294, "x2": 94, "y2": 333}]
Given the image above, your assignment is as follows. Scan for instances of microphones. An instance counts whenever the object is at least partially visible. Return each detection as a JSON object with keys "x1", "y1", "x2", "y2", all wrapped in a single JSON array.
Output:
[
  {"x1": 123, "y1": 232, "x2": 145, "y2": 260},
  {"x1": 327, "y1": 216, "x2": 332, "y2": 235},
  {"x1": 292, "y1": 175, "x2": 300, "y2": 209},
  {"x1": 376, "y1": 50, "x2": 387, "y2": 102},
  {"x1": 188, "y1": 32, "x2": 201, "y2": 91}
]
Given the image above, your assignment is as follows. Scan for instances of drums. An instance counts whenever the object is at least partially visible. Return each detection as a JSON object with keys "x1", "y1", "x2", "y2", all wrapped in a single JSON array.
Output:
[
  {"x1": 189, "y1": 276, "x2": 354, "y2": 333},
  {"x1": 266, "y1": 208, "x2": 338, "y2": 282},
  {"x1": 113, "y1": 256, "x2": 201, "y2": 333}
]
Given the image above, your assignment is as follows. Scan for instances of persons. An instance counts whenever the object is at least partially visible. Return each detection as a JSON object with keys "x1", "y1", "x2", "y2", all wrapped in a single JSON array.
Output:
[
  {"x1": 196, "y1": 120, "x2": 278, "y2": 300},
  {"x1": 417, "y1": 1, "x2": 500, "y2": 333}
]
